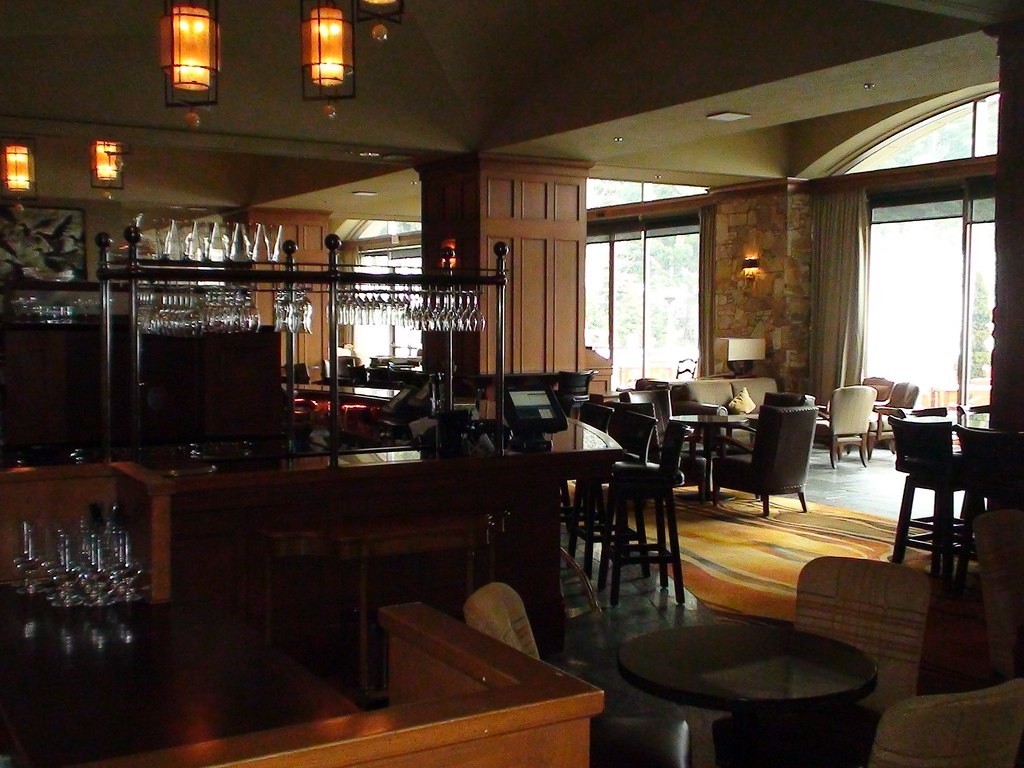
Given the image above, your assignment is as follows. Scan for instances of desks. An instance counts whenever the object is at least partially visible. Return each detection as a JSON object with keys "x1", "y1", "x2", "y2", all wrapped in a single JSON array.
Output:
[
  {"x1": 618, "y1": 621, "x2": 880, "y2": 767},
  {"x1": 0, "y1": 568, "x2": 364, "y2": 768},
  {"x1": 668, "y1": 413, "x2": 750, "y2": 502}
]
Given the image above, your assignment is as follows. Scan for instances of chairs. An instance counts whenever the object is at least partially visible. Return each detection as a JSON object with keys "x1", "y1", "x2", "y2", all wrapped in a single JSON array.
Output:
[
  {"x1": 603, "y1": 375, "x2": 820, "y2": 614},
  {"x1": 861, "y1": 378, "x2": 920, "y2": 460},
  {"x1": 816, "y1": 385, "x2": 879, "y2": 469},
  {"x1": 710, "y1": 556, "x2": 933, "y2": 768},
  {"x1": 865, "y1": 678, "x2": 1024, "y2": 768},
  {"x1": 281, "y1": 354, "x2": 425, "y2": 388},
  {"x1": 463, "y1": 577, "x2": 691, "y2": 768},
  {"x1": 972, "y1": 509, "x2": 1024, "y2": 679}
]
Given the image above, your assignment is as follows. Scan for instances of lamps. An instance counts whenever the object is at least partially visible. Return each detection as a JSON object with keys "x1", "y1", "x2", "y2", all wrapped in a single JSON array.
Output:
[
  {"x1": 89, "y1": 137, "x2": 126, "y2": 199},
  {"x1": 1, "y1": 143, "x2": 37, "y2": 214},
  {"x1": 154, "y1": 0, "x2": 228, "y2": 109},
  {"x1": 294, "y1": 0, "x2": 359, "y2": 120},
  {"x1": 727, "y1": 339, "x2": 766, "y2": 379}
]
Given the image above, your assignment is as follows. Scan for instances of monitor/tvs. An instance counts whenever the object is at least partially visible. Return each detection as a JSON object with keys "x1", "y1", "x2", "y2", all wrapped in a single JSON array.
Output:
[
  {"x1": 382, "y1": 384, "x2": 419, "y2": 415},
  {"x1": 503, "y1": 384, "x2": 568, "y2": 432}
]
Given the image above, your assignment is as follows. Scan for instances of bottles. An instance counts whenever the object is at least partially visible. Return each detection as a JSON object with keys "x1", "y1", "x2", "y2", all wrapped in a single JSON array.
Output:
[
  {"x1": 11, "y1": 520, "x2": 148, "y2": 607},
  {"x1": 468, "y1": 423, "x2": 496, "y2": 457},
  {"x1": 164, "y1": 219, "x2": 283, "y2": 262}
]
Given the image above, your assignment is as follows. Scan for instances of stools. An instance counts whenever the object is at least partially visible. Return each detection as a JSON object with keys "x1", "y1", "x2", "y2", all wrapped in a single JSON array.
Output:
[
  {"x1": 954, "y1": 423, "x2": 1024, "y2": 592},
  {"x1": 552, "y1": 369, "x2": 685, "y2": 607},
  {"x1": 888, "y1": 413, "x2": 986, "y2": 576}
]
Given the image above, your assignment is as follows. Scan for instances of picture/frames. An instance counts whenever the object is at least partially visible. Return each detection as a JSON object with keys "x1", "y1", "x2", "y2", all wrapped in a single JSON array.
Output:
[{"x1": 0, "y1": 204, "x2": 87, "y2": 283}]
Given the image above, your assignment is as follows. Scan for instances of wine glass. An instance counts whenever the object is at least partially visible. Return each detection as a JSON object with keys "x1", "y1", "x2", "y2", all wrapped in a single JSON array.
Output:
[
  {"x1": 273, "y1": 287, "x2": 312, "y2": 334},
  {"x1": 203, "y1": 286, "x2": 260, "y2": 332},
  {"x1": 325, "y1": 288, "x2": 485, "y2": 332},
  {"x1": 136, "y1": 283, "x2": 199, "y2": 334}
]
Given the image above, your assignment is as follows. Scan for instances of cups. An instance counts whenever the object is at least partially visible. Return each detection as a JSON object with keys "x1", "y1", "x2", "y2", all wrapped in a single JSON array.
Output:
[{"x1": 39, "y1": 304, "x2": 76, "y2": 323}]
